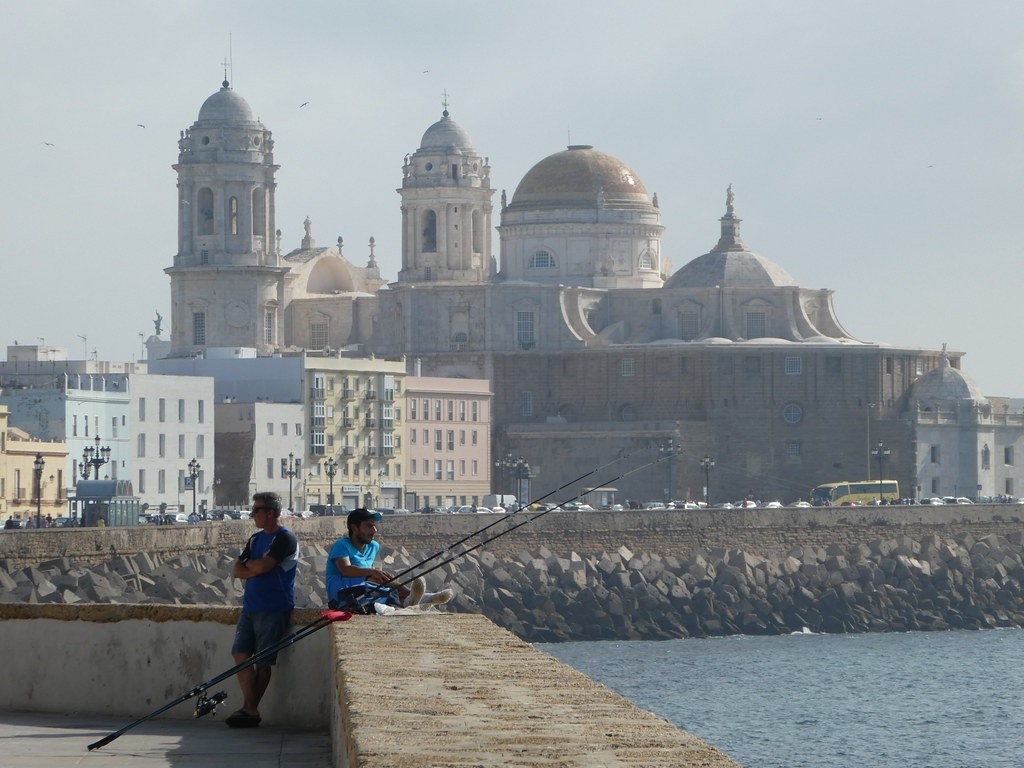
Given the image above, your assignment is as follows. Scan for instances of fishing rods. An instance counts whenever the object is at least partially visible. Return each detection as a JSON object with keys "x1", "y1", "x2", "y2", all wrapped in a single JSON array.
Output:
[{"x1": 85, "y1": 444, "x2": 681, "y2": 753}]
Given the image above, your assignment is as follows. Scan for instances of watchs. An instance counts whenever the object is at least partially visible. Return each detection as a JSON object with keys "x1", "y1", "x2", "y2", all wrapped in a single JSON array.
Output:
[{"x1": 243, "y1": 558, "x2": 249, "y2": 566}]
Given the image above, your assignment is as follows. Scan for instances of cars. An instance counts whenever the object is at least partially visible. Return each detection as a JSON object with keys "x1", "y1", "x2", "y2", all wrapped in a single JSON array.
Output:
[
  {"x1": 422, "y1": 501, "x2": 561, "y2": 513},
  {"x1": 734, "y1": 496, "x2": 975, "y2": 509},
  {"x1": 0, "y1": 515, "x2": 81, "y2": 529},
  {"x1": 563, "y1": 501, "x2": 733, "y2": 510},
  {"x1": 138, "y1": 510, "x2": 313, "y2": 523}
]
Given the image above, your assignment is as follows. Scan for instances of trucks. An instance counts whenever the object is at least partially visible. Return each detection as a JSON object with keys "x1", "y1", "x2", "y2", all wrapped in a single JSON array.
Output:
[{"x1": 310, "y1": 504, "x2": 349, "y2": 516}]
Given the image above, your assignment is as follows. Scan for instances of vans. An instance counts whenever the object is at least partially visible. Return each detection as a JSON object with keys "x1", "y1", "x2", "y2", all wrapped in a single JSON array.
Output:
[{"x1": 482, "y1": 495, "x2": 516, "y2": 509}]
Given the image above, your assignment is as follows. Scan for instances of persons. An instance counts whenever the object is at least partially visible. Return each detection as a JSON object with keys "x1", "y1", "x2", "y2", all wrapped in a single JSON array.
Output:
[
  {"x1": 155, "y1": 514, "x2": 172, "y2": 525},
  {"x1": 328, "y1": 507, "x2": 335, "y2": 516},
  {"x1": 326, "y1": 507, "x2": 453, "y2": 612},
  {"x1": 821, "y1": 496, "x2": 915, "y2": 507},
  {"x1": 742, "y1": 498, "x2": 748, "y2": 507},
  {"x1": 500, "y1": 499, "x2": 519, "y2": 513},
  {"x1": 4, "y1": 516, "x2": 13, "y2": 529},
  {"x1": 988, "y1": 493, "x2": 1012, "y2": 504},
  {"x1": 755, "y1": 497, "x2": 761, "y2": 507},
  {"x1": 96, "y1": 516, "x2": 105, "y2": 527},
  {"x1": 624, "y1": 499, "x2": 630, "y2": 509},
  {"x1": 225, "y1": 492, "x2": 301, "y2": 727},
  {"x1": 24, "y1": 513, "x2": 85, "y2": 528},
  {"x1": 188, "y1": 511, "x2": 207, "y2": 526},
  {"x1": 471, "y1": 503, "x2": 477, "y2": 512}
]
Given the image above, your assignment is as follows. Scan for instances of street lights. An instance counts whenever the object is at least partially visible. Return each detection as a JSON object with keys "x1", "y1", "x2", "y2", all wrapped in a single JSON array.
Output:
[
  {"x1": 282, "y1": 453, "x2": 300, "y2": 513},
  {"x1": 34, "y1": 452, "x2": 45, "y2": 527},
  {"x1": 513, "y1": 455, "x2": 528, "y2": 511},
  {"x1": 79, "y1": 435, "x2": 112, "y2": 505},
  {"x1": 324, "y1": 457, "x2": 338, "y2": 516},
  {"x1": 700, "y1": 455, "x2": 714, "y2": 508},
  {"x1": 188, "y1": 458, "x2": 200, "y2": 512},
  {"x1": 495, "y1": 452, "x2": 510, "y2": 508},
  {"x1": 872, "y1": 439, "x2": 891, "y2": 501},
  {"x1": 660, "y1": 436, "x2": 681, "y2": 503}
]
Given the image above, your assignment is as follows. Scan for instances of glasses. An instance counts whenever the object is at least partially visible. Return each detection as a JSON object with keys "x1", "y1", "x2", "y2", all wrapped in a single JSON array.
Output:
[{"x1": 252, "y1": 506, "x2": 268, "y2": 514}]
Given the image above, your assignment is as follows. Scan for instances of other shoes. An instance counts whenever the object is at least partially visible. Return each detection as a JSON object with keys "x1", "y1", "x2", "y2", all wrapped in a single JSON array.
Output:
[{"x1": 224, "y1": 709, "x2": 262, "y2": 728}]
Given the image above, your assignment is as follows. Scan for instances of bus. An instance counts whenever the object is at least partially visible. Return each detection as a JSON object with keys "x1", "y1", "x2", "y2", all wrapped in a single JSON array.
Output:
[{"x1": 810, "y1": 479, "x2": 899, "y2": 506}]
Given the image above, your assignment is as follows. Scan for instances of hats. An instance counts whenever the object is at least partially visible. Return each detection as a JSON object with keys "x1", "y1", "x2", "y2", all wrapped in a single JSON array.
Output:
[{"x1": 347, "y1": 508, "x2": 383, "y2": 526}]
